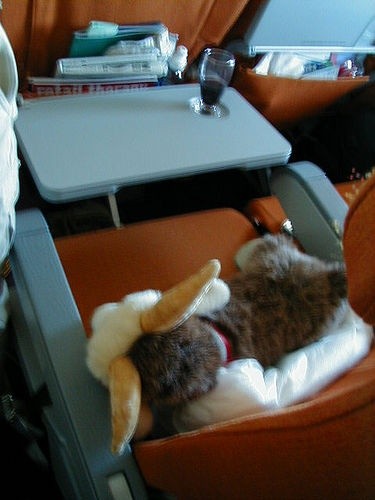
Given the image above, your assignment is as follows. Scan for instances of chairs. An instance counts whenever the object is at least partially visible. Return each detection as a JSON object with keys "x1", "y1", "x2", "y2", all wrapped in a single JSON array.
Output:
[{"x1": 9, "y1": 160, "x2": 375, "y2": 500}]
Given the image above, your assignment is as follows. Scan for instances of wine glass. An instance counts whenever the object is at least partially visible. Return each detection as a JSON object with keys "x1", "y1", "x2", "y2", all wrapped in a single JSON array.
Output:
[{"x1": 189, "y1": 47, "x2": 236, "y2": 120}]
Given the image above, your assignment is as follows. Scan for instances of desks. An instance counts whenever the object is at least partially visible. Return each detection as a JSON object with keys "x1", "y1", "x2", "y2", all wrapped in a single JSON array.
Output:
[{"x1": 13, "y1": 83, "x2": 292, "y2": 206}]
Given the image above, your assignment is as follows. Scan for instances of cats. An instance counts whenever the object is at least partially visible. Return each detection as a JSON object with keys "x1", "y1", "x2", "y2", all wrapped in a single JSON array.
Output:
[{"x1": 130, "y1": 232, "x2": 349, "y2": 412}]
